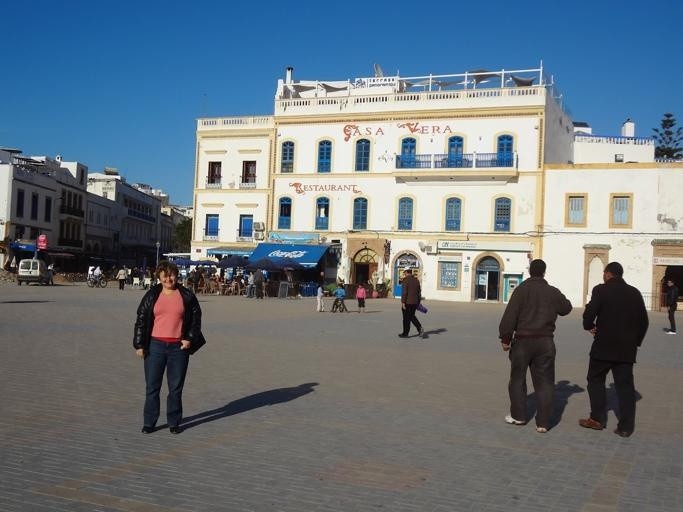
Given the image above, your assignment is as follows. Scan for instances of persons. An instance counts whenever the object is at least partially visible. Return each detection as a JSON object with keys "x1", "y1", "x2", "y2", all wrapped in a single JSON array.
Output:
[
  {"x1": 578, "y1": 260, "x2": 649, "y2": 437},
  {"x1": 664, "y1": 279, "x2": 679, "y2": 335},
  {"x1": 499, "y1": 258, "x2": 571, "y2": 432},
  {"x1": 93, "y1": 264, "x2": 103, "y2": 288},
  {"x1": 397, "y1": 269, "x2": 425, "y2": 338},
  {"x1": 46, "y1": 261, "x2": 55, "y2": 284},
  {"x1": 132, "y1": 262, "x2": 204, "y2": 434},
  {"x1": 314, "y1": 282, "x2": 367, "y2": 314},
  {"x1": 115, "y1": 266, "x2": 127, "y2": 290},
  {"x1": 187, "y1": 264, "x2": 269, "y2": 299}
]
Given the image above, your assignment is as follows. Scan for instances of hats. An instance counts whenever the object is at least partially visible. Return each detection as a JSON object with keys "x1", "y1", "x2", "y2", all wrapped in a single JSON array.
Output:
[{"x1": 403, "y1": 266, "x2": 412, "y2": 273}]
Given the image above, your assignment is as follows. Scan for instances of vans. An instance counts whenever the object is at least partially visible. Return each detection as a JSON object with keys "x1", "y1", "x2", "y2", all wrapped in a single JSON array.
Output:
[{"x1": 16, "y1": 259, "x2": 49, "y2": 286}]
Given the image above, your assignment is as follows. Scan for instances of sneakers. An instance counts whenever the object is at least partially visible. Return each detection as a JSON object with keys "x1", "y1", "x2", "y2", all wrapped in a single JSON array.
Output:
[
  {"x1": 580, "y1": 419, "x2": 603, "y2": 430},
  {"x1": 399, "y1": 333, "x2": 408, "y2": 338},
  {"x1": 505, "y1": 415, "x2": 547, "y2": 432},
  {"x1": 419, "y1": 327, "x2": 424, "y2": 337}
]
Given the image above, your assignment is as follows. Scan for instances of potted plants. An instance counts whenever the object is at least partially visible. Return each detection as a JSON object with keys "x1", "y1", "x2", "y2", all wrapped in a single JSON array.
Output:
[
  {"x1": 366, "y1": 271, "x2": 379, "y2": 298},
  {"x1": 379, "y1": 269, "x2": 392, "y2": 298}
]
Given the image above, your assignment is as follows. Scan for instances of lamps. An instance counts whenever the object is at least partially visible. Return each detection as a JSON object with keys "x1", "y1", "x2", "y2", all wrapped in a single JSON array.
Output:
[{"x1": 614, "y1": 153, "x2": 623, "y2": 163}]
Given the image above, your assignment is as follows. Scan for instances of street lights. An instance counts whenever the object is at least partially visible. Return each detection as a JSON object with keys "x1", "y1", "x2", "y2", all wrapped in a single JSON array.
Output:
[{"x1": 155, "y1": 241, "x2": 161, "y2": 268}]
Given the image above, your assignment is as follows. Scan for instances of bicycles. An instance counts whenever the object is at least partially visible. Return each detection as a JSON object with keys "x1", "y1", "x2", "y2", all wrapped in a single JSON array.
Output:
[
  {"x1": 87, "y1": 274, "x2": 108, "y2": 288},
  {"x1": 332, "y1": 298, "x2": 344, "y2": 313}
]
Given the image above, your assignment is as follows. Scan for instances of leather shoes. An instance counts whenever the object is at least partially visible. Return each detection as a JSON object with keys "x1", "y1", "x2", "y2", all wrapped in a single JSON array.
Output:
[
  {"x1": 170, "y1": 426, "x2": 180, "y2": 434},
  {"x1": 142, "y1": 426, "x2": 153, "y2": 433}
]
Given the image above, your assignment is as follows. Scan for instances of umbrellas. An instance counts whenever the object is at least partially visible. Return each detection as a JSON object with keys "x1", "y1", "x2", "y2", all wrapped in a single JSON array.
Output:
[{"x1": 173, "y1": 255, "x2": 249, "y2": 268}]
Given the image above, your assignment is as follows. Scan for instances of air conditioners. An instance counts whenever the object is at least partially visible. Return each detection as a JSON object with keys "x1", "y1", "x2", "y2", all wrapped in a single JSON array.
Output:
[
  {"x1": 254, "y1": 231, "x2": 263, "y2": 240},
  {"x1": 253, "y1": 221, "x2": 264, "y2": 232},
  {"x1": 425, "y1": 245, "x2": 437, "y2": 255}
]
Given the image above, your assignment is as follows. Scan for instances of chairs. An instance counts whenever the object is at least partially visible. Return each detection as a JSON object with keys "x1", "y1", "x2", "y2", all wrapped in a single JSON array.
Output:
[
  {"x1": 195, "y1": 276, "x2": 321, "y2": 296},
  {"x1": 131, "y1": 277, "x2": 160, "y2": 289}
]
